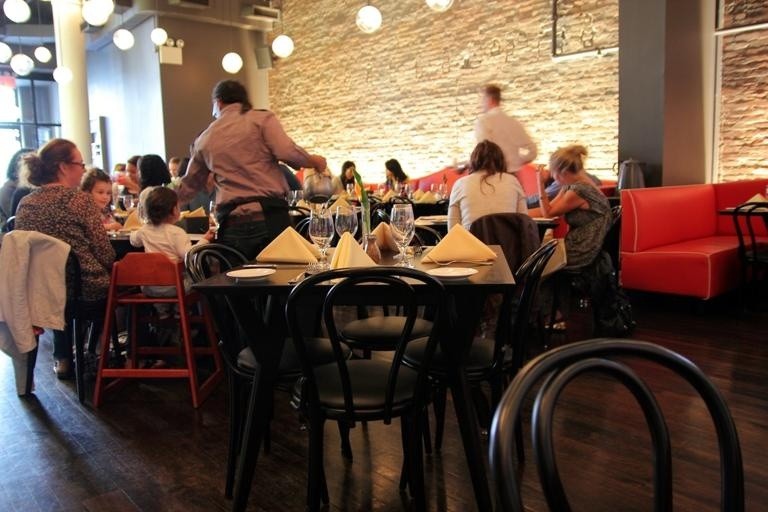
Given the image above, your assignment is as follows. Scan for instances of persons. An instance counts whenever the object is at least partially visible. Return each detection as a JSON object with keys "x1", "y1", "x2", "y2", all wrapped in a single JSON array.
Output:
[
  {"x1": 383, "y1": 158, "x2": 411, "y2": 187},
  {"x1": 173, "y1": 79, "x2": 326, "y2": 319},
  {"x1": 528, "y1": 144, "x2": 615, "y2": 332},
  {"x1": 0, "y1": 139, "x2": 215, "y2": 379},
  {"x1": 452, "y1": 82, "x2": 540, "y2": 176},
  {"x1": 331, "y1": 160, "x2": 356, "y2": 200},
  {"x1": 446, "y1": 138, "x2": 528, "y2": 338},
  {"x1": 528, "y1": 173, "x2": 604, "y2": 208}
]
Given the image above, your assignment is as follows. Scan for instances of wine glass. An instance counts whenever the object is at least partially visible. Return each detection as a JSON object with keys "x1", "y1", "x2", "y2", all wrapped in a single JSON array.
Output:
[
  {"x1": 345, "y1": 183, "x2": 450, "y2": 202},
  {"x1": 287, "y1": 191, "x2": 295, "y2": 205},
  {"x1": 309, "y1": 209, "x2": 335, "y2": 272},
  {"x1": 123, "y1": 195, "x2": 135, "y2": 211},
  {"x1": 392, "y1": 204, "x2": 415, "y2": 259},
  {"x1": 335, "y1": 206, "x2": 359, "y2": 240},
  {"x1": 210, "y1": 201, "x2": 220, "y2": 231},
  {"x1": 391, "y1": 209, "x2": 416, "y2": 268},
  {"x1": 294, "y1": 190, "x2": 304, "y2": 203}
]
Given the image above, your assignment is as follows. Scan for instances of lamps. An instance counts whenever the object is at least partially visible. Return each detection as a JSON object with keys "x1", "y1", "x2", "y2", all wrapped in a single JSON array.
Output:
[
  {"x1": 220, "y1": 0, "x2": 244, "y2": 75},
  {"x1": 424, "y1": 0, "x2": 452, "y2": 13},
  {"x1": 0, "y1": 0, "x2": 167, "y2": 76},
  {"x1": 355, "y1": 0, "x2": 384, "y2": 34},
  {"x1": 272, "y1": 0, "x2": 294, "y2": 58}
]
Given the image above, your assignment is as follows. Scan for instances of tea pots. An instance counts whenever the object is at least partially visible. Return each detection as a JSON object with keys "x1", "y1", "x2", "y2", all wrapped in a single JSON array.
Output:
[
  {"x1": 304, "y1": 166, "x2": 333, "y2": 202},
  {"x1": 613, "y1": 157, "x2": 648, "y2": 194}
]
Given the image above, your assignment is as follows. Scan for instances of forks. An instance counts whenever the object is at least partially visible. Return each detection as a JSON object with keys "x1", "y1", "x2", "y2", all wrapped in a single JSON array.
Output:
[{"x1": 428, "y1": 256, "x2": 494, "y2": 267}]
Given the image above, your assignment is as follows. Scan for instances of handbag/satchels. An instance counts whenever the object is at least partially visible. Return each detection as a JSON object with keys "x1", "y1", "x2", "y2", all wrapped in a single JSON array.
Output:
[{"x1": 592, "y1": 271, "x2": 637, "y2": 336}]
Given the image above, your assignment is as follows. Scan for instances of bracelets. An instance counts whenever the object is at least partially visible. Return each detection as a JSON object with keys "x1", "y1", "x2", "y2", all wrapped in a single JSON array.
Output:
[{"x1": 539, "y1": 195, "x2": 548, "y2": 200}]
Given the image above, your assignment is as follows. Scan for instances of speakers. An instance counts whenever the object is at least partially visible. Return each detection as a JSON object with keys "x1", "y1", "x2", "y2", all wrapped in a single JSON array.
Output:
[{"x1": 254, "y1": 46, "x2": 273, "y2": 71}]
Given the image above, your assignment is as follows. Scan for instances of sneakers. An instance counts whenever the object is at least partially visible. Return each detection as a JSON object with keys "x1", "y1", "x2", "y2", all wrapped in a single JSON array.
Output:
[
  {"x1": 175, "y1": 311, "x2": 192, "y2": 319},
  {"x1": 53, "y1": 361, "x2": 77, "y2": 379},
  {"x1": 159, "y1": 311, "x2": 171, "y2": 319}
]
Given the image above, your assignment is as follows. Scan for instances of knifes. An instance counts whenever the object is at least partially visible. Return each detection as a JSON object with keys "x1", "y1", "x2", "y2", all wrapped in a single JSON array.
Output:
[{"x1": 242, "y1": 264, "x2": 308, "y2": 269}]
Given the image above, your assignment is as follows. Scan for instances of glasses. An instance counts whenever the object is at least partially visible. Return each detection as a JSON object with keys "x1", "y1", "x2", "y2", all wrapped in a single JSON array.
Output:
[{"x1": 67, "y1": 159, "x2": 85, "y2": 168}]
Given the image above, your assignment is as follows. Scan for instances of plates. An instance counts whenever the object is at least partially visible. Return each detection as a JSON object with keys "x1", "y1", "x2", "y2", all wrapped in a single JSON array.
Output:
[
  {"x1": 427, "y1": 268, "x2": 479, "y2": 281},
  {"x1": 227, "y1": 269, "x2": 276, "y2": 281}
]
